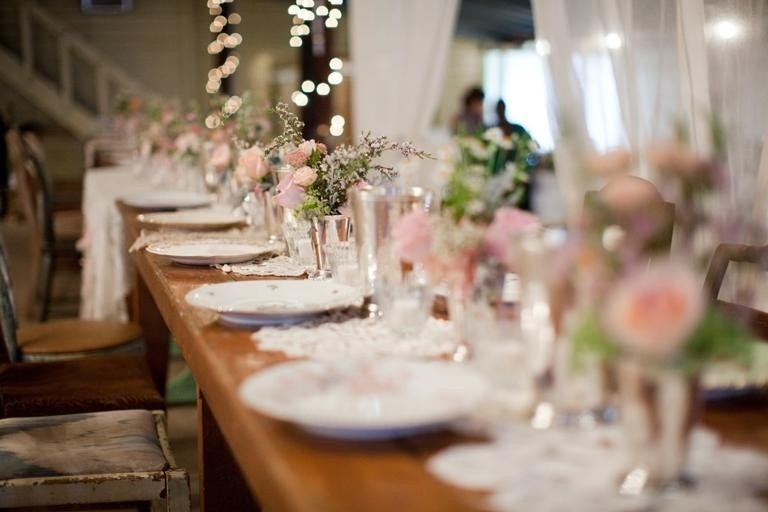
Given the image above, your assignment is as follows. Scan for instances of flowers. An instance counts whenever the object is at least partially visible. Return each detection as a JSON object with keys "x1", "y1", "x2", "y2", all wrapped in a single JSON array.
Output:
[{"x1": 261, "y1": 97, "x2": 440, "y2": 222}]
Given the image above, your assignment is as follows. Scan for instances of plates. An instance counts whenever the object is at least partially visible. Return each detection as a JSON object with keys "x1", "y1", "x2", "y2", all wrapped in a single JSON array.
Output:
[
  {"x1": 246, "y1": 318, "x2": 453, "y2": 358},
  {"x1": 140, "y1": 241, "x2": 276, "y2": 271},
  {"x1": 119, "y1": 191, "x2": 209, "y2": 209},
  {"x1": 179, "y1": 279, "x2": 358, "y2": 326},
  {"x1": 139, "y1": 210, "x2": 245, "y2": 231},
  {"x1": 417, "y1": 425, "x2": 713, "y2": 498},
  {"x1": 231, "y1": 358, "x2": 477, "y2": 445}
]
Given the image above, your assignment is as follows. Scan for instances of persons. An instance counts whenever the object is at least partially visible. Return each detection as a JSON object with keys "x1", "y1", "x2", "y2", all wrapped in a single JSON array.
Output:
[
  {"x1": 487, "y1": 96, "x2": 534, "y2": 144},
  {"x1": 450, "y1": 85, "x2": 491, "y2": 146}
]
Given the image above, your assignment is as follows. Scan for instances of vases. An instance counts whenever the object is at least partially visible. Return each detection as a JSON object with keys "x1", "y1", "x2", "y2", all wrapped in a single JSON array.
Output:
[{"x1": 315, "y1": 214, "x2": 351, "y2": 279}]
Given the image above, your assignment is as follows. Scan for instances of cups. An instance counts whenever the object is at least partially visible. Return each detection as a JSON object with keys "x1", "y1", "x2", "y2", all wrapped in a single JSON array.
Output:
[
  {"x1": 457, "y1": 271, "x2": 695, "y2": 479},
  {"x1": 351, "y1": 184, "x2": 428, "y2": 306},
  {"x1": 270, "y1": 164, "x2": 307, "y2": 259},
  {"x1": 310, "y1": 215, "x2": 351, "y2": 270},
  {"x1": 202, "y1": 153, "x2": 275, "y2": 228},
  {"x1": 321, "y1": 241, "x2": 356, "y2": 281}
]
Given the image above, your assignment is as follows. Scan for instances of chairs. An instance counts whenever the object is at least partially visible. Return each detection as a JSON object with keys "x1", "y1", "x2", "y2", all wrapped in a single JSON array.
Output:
[
  {"x1": 4, "y1": 127, "x2": 90, "y2": 319},
  {"x1": 1, "y1": 245, "x2": 149, "y2": 405},
  {"x1": 581, "y1": 174, "x2": 676, "y2": 279},
  {"x1": 0, "y1": 406, "x2": 191, "y2": 511},
  {"x1": 698, "y1": 244, "x2": 768, "y2": 342}
]
